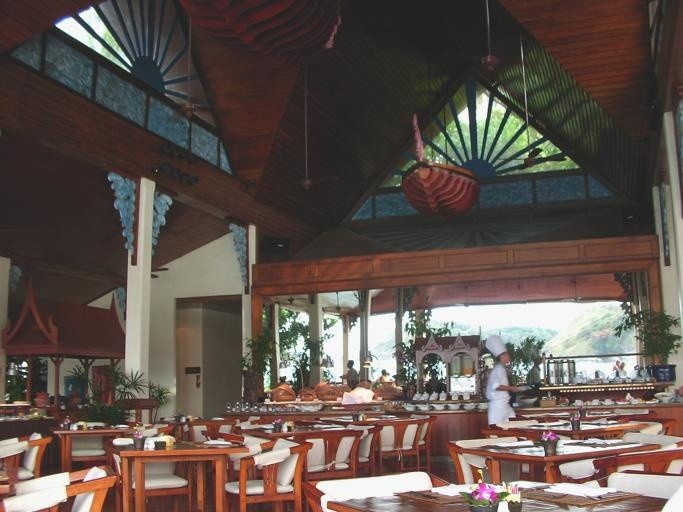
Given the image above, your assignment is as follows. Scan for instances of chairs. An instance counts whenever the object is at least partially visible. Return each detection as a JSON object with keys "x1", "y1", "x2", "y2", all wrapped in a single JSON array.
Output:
[{"x1": 0, "y1": 399, "x2": 683, "y2": 512}]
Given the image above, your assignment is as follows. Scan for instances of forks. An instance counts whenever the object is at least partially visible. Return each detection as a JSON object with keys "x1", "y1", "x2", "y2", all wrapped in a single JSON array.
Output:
[
  {"x1": 405, "y1": 488, "x2": 438, "y2": 500},
  {"x1": 232, "y1": 414, "x2": 430, "y2": 434},
  {"x1": 517, "y1": 480, "x2": 630, "y2": 501},
  {"x1": 479, "y1": 409, "x2": 650, "y2": 453}
]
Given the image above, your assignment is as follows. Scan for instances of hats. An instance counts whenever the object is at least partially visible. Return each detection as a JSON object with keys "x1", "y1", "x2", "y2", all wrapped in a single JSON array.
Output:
[{"x1": 485, "y1": 336, "x2": 507, "y2": 356}]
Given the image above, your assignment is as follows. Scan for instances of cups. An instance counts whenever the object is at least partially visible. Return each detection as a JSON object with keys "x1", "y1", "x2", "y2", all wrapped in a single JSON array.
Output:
[
  {"x1": 264, "y1": 398, "x2": 271, "y2": 403},
  {"x1": 313, "y1": 397, "x2": 320, "y2": 402},
  {"x1": 337, "y1": 397, "x2": 342, "y2": 401},
  {"x1": 377, "y1": 396, "x2": 383, "y2": 402},
  {"x1": 143, "y1": 435, "x2": 177, "y2": 450},
  {"x1": 537, "y1": 368, "x2": 658, "y2": 387}
]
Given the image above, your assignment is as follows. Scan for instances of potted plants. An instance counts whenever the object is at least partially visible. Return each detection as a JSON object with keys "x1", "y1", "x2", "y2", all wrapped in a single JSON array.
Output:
[
  {"x1": 391, "y1": 339, "x2": 417, "y2": 399},
  {"x1": 239, "y1": 327, "x2": 277, "y2": 402},
  {"x1": 614, "y1": 309, "x2": 683, "y2": 380}
]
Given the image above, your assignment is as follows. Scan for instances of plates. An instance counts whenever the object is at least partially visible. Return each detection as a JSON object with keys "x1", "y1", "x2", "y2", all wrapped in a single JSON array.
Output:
[
  {"x1": 112, "y1": 437, "x2": 135, "y2": 449},
  {"x1": 204, "y1": 439, "x2": 233, "y2": 451},
  {"x1": 158, "y1": 414, "x2": 225, "y2": 424},
  {"x1": 511, "y1": 386, "x2": 682, "y2": 406},
  {"x1": 514, "y1": 383, "x2": 536, "y2": 393},
  {"x1": 0, "y1": 391, "x2": 153, "y2": 430}
]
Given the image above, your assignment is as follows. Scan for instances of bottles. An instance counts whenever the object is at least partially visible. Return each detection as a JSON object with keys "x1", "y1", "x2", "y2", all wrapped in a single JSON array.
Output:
[
  {"x1": 388, "y1": 389, "x2": 490, "y2": 412},
  {"x1": 222, "y1": 399, "x2": 323, "y2": 414},
  {"x1": 132, "y1": 427, "x2": 146, "y2": 451},
  {"x1": 267, "y1": 390, "x2": 272, "y2": 402},
  {"x1": 296, "y1": 395, "x2": 301, "y2": 404}
]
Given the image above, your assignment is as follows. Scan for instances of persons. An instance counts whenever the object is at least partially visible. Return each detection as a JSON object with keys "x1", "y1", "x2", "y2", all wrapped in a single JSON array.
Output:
[
  {"x1": 614, "y1": 361, "x2": 620, "y2": 377},
  {"x1": 346, "y1": 360, "x2": 358, "y2": 390},
  {"x1": 381, "y1": 370, "x2": 389, "y2": 383},
  {"x1": 485, "y1": 335, "x2": 520, "y2": 431},
  {"x1": 620, "y1": 362, "x2": 628, "y2": 376},
  {"x1": 526, "y1": 351, "x2": 543, "y2": 386}
]
